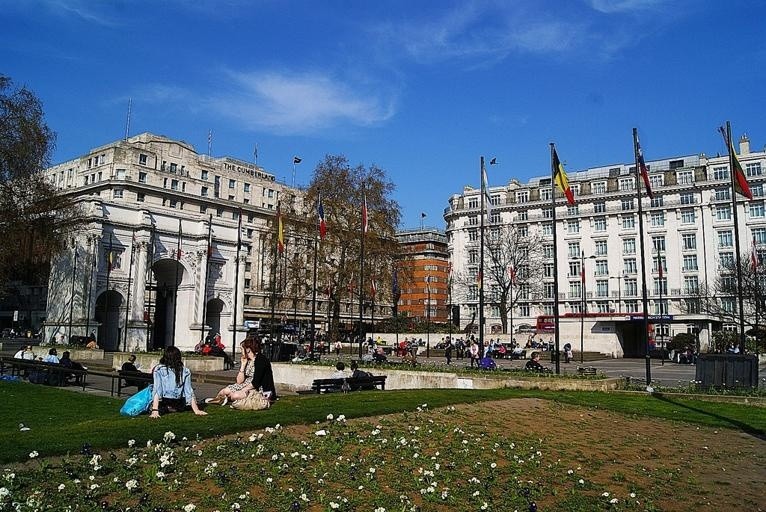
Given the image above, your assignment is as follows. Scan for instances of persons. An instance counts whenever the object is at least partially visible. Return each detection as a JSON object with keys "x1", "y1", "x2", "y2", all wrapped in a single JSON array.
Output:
[
  {"x1": 204, "y1": 339, "x2": 277, "y2": 409},
  {"x1": 329, "y1": 363, "x2": 350, "y2": 392},
  {"x1": 1, "y1": 322, "x2": 99, "y2": 378},
  {"x1": 121, "y1": 355, "x2": 144, "y2": 373},
  {"x1": 194, "y1": 330, "x2": 226, "y2": 356},
  {"x1": 280, "y1": 328, "x2": 575, "y2": 373},
  {"x1": 148, "y1": 345, "x2": 209, "y2": 420}
]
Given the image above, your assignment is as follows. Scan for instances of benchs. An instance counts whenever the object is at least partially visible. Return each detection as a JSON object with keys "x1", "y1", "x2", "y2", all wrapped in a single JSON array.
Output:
[
  {"x1": 295, "y1": 374, "x2": 389, "y2": 398},
  {"x1": 0, "y1": 355, "x2": 154, "y2": 397}
]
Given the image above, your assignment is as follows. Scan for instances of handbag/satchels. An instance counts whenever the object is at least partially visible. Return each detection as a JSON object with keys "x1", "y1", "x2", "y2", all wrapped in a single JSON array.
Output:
[
  {"x1": 232, "y1": 389, "x2": 270, "y2": 411},
  {"x1": 119, "y1": 384, "x2": 154, "y2": 418}
]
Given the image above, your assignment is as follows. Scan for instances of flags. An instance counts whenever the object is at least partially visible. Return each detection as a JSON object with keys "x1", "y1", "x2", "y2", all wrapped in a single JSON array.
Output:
[
  {"x1": 553, "y1": 145, "x2": 578, "y2": 208},
  {"x1": 636, "y1": 132, "x2": 656, "y2": 201},
  {"x1": 278, "y1": 210, "x2": 286, "y2": 257},
  {"x1": 363, "y1": 188, "x2": 370, "y2": 234},
  {"x1": 721, "y1": 127, "x2": 755, "y2": 202},
  {"x1": 484, "y1": 169, "x2": 495, "y2": 221},
  {"x1": 319, "y1": 196, "x2": 329, "y2": 239}
]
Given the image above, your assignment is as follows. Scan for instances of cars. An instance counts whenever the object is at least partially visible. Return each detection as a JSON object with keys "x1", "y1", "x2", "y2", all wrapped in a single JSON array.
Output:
[
  {"x1": 388, "y1": 358, "x2": 421, "y2": 369},
  {"x1": 362, "y1": 354, "x2": 387, "y2": 364}
]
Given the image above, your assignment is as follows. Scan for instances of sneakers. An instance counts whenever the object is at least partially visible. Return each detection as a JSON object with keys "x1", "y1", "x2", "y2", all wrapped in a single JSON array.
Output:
[{"x1": 205, "y1": 395, "x2": 228, "y2": 407}]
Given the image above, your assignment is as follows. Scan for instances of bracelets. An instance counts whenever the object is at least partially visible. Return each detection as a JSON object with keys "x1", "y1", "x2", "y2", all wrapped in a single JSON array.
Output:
[{"x1": 149, "y1": 408, "x2": 160, "y2": 412}]
